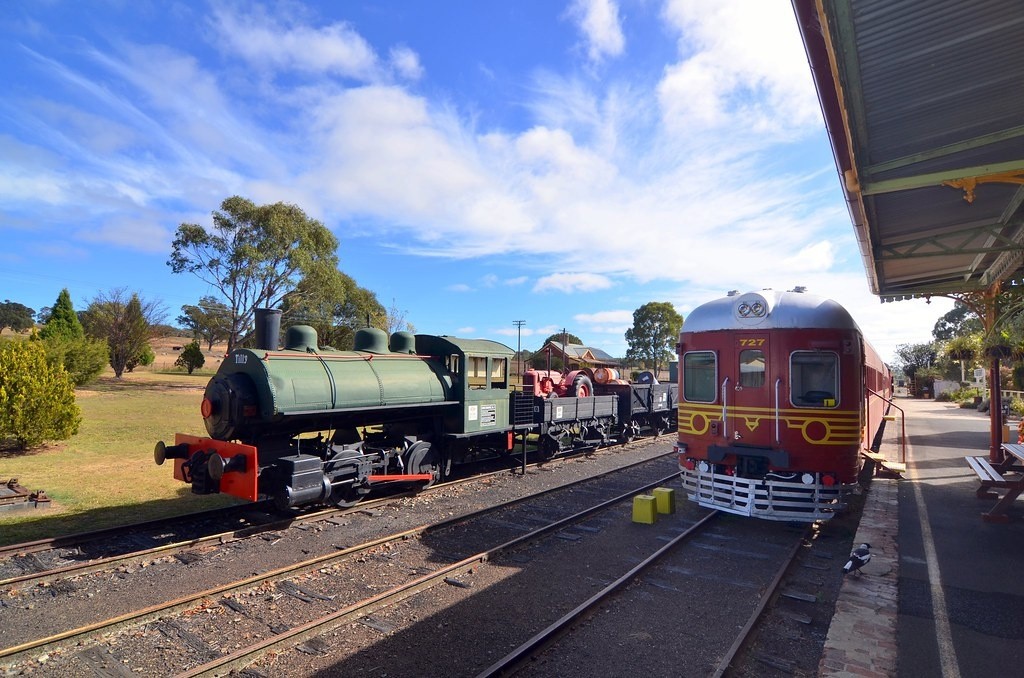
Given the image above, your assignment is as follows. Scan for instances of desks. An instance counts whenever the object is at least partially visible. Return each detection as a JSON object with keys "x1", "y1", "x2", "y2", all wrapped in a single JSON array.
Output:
[{"x1": 976, "y1": 444, "x2": 1024, "y2": 523}]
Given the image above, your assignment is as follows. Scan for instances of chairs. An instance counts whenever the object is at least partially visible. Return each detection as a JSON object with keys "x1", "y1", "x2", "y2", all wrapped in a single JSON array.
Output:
[{"x1": 802, "y1": 391, "x2": 832, "y2": 403}]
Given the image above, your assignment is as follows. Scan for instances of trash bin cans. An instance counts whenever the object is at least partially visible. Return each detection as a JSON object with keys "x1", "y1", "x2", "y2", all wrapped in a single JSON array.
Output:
[{"x1": 922, "y1": 386, "x2": 930, "y2": 399}]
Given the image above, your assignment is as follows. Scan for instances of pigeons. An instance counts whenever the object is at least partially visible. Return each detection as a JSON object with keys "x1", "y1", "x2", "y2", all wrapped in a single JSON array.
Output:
[{"x1": 843, "y1": 543, "x2": 872, "y2": 576}]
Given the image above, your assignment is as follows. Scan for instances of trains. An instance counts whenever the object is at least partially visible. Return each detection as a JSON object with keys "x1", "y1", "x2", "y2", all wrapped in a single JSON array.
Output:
[
  {"x1": 676, "y1": 285, "x2": 894, "y2": 522},
  {"x1": 154, "y1": 308, "x2": 679, "y2": 509}
]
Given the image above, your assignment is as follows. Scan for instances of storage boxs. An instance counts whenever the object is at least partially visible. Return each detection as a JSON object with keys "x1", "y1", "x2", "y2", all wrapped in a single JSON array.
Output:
[
  {"x1": 653, "y1": 487, "x2": 676, "y2": 515},
  {"x1": 632, "y1": 495, "x2": 656, "y2": 524}
]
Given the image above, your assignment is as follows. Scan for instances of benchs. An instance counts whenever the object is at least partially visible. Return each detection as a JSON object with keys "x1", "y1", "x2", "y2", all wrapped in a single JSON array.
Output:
[{"x1": 965, "y1": 456, "x2": 1024, "y2": 488}]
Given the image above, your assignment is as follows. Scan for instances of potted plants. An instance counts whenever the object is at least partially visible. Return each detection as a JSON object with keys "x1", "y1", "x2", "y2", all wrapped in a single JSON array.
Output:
[{"x1": 943, "y1": 328, "x2": 1024, "y2": 364}]
